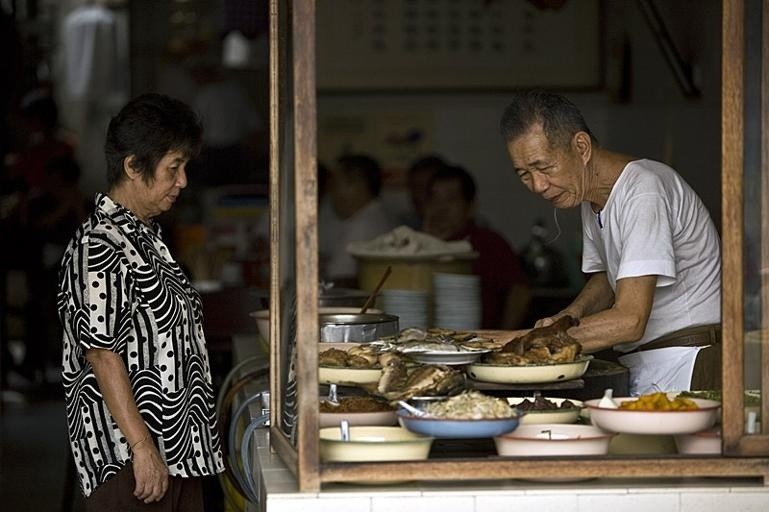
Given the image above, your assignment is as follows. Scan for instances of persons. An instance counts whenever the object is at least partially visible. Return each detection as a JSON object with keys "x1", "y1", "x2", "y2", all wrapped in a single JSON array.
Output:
[
  {"x1": 317, "y1": 150, "x2": 524, "y2": 317},
  {"x1": 55, "y1": 91, "x2": 226, "y2": 512},
  {"x1": 500, "y1": 89, "x2": 722, "y2": 397}
]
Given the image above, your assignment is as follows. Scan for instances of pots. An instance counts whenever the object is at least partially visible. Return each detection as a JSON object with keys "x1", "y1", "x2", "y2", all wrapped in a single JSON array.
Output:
[
  {"x1": 349, "y1": 252, "x2": 479, "y2": 294},
  {"x1": 319, "y1": 284, "x2": 376, "y2": 309},
  {"x1": 318, "y1": 314, "x2": 401, "y2": 344}
]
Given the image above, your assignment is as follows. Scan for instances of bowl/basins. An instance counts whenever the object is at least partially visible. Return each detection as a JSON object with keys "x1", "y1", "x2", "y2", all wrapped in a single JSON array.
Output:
[
  {"x1": 318, "y1": 425, "x2": 438, "y2": 463},
  {"x1": 248, "y1": 306, "x2": 391, "y2": 347},
  {"x1": 505, "y1": 396, "x2": 586, "y2": 422},
  {"x1": 490, "y1": 422, "x2": 621, "y2": 456},
  {"x1": 318, "y1": 396, "x2": 400, "y2": 426}
]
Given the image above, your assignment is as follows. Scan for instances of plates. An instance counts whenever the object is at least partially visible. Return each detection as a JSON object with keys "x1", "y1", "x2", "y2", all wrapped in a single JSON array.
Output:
[
  {"x1": 380, "y1": 289, "x2": 432, "y2": 329},
  {"x1": 395, "y1": 407, "x2": 521, "y2": 440},
  {"x1": 464, "y1": 355, "x2": 595, "y2": 383},
  {"x1": 390, "y1": 347, "x2": 491, "y2": 366},
  {"x1": 586, "y1": 388, "x2": 761, "y2": 455},
  {"x1": 316, "y1": 365, "x2": 384, "y2": 388},
  {"x1": 430, "y1": 271, "x2": 483, "y2": 330}
]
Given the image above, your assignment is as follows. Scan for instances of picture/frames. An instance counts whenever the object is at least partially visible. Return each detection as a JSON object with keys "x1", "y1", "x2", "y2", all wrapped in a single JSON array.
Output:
[{"x1": 315, "y1": 1, "x2": 607, "y2": 97}]
